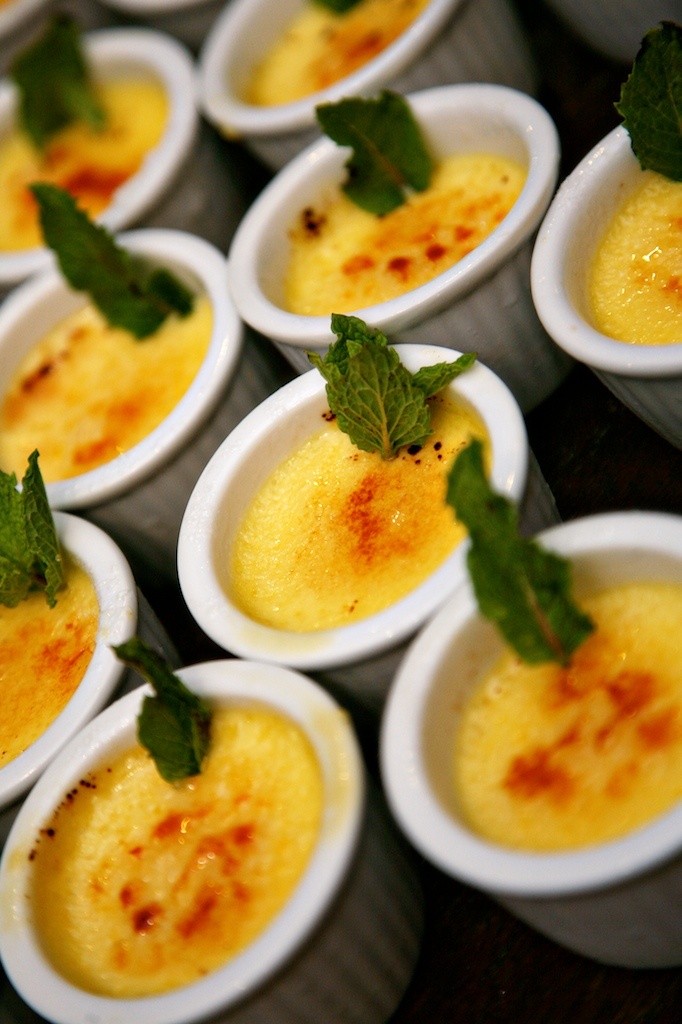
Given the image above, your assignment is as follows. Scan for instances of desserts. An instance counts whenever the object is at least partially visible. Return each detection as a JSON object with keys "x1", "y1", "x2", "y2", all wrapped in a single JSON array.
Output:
[{"x1": 0, "y1": 0, "x2": 682, "y2": 1024}]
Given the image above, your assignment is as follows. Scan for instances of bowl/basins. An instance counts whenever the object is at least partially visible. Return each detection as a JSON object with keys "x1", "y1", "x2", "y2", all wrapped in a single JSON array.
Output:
[
  {"x1": 226, "y1": 83, "x2": 562, "y2": 420},
  {"x1": 0, "y1": 1, "x2": 529, "y2": 278},
  {"x1": 0, "y1": 227, "x2": 268, "y2": 576},
  {"x1": 1, "y1": 658, "x2": 430, "y2": 1023},
  {"x1": 380, "y1": 511, "x2": 682, "y2": 965},
  {"x1": 0, "y1": 512, "x2": 175, "y2": 830},
  {"x1": 176, "y1": 342, "x2": 563, "y2": 696},
  {"x1": 528, "y1": 118, "x2": 682, "y2": 448}
]
